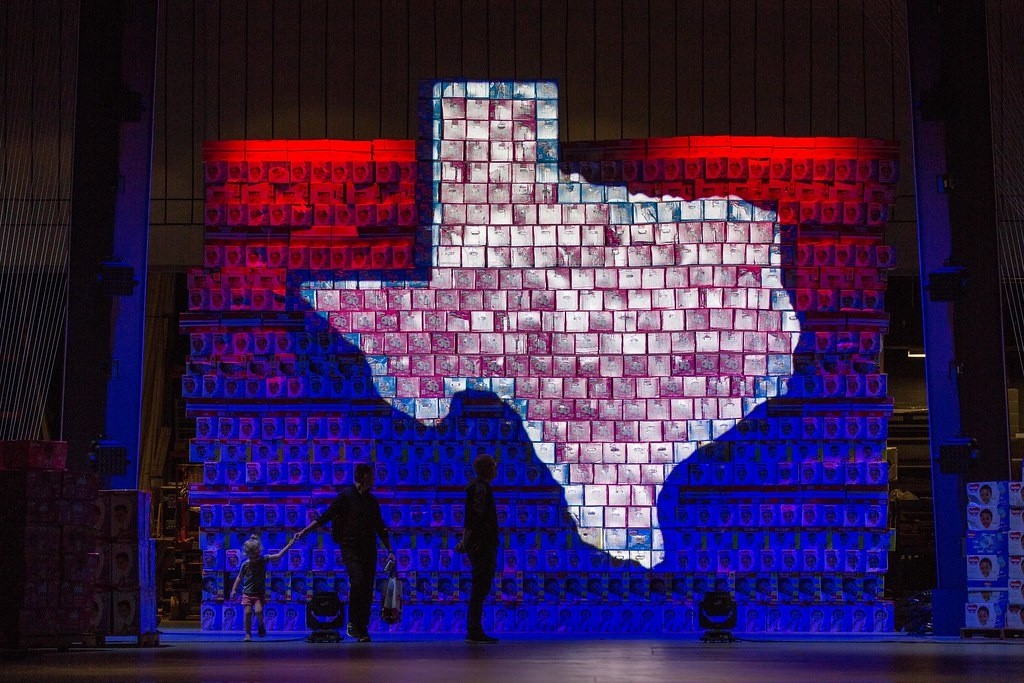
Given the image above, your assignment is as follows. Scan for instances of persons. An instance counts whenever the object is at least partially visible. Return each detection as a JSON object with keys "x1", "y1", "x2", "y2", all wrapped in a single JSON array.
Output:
[
  {"x1": 980, "y1": 485, "x2": 992, "y2": 504},
  {"x1": 977, "y1": 606, "x2": 989, "y2": 625},
  {"x1": 979, "y1": 557, "x2": 992, "y2": 577},
  {"x1": 982, "y1": 591, "x2": 992, "y2": 600},
  {"x1": 454, "y1": 455, "x2": 500, "y2": 644},
  {"x1": 117, "y1": 600, "x2": 133, "y2": 624},
  {"x1": 114, "y1": 505, "x2": 131, "y2": 532},
  {"x1": 115, "y1": 552, "x2": 130, "y2": 575},
  {"x1": 230, "y1": 536, "x2": 295, "y2": 641},
  {"x1": 980, "y1": 509, "x2": 993, "y2": 528},
  {"x1": 294, "y1": 464, "x2": 396, "y2": 641}
]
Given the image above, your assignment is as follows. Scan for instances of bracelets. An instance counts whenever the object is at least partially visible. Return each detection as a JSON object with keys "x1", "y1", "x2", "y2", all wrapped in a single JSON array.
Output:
[{"x1": 388, "y1": 550, "x2": 394, "y2": 554}]
